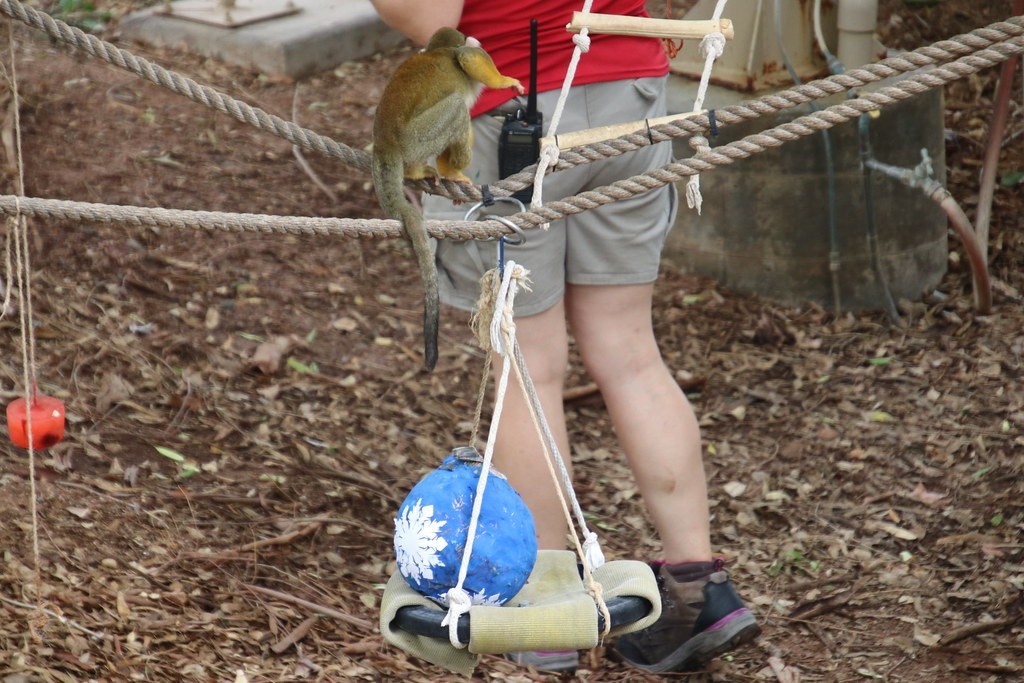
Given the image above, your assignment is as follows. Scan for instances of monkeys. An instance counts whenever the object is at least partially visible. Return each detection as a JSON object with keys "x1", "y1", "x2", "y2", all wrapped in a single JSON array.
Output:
[{"x1": 371, "y1": 27, "x2": 526, "y2": 381}]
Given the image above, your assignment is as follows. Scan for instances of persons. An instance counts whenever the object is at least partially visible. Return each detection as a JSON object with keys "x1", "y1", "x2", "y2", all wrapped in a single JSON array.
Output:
[{"x1": 371, "y1": 0, "x2": 761, "y2": 671}]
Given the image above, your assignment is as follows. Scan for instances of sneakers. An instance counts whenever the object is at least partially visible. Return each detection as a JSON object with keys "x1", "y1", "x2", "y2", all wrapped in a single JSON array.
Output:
[
  {"x1": 507, "y1": 563, "x2": 583, "y2": 677},
  {"x1": 647, "y1": 560, "x2": 762, "y2": 672}
]
